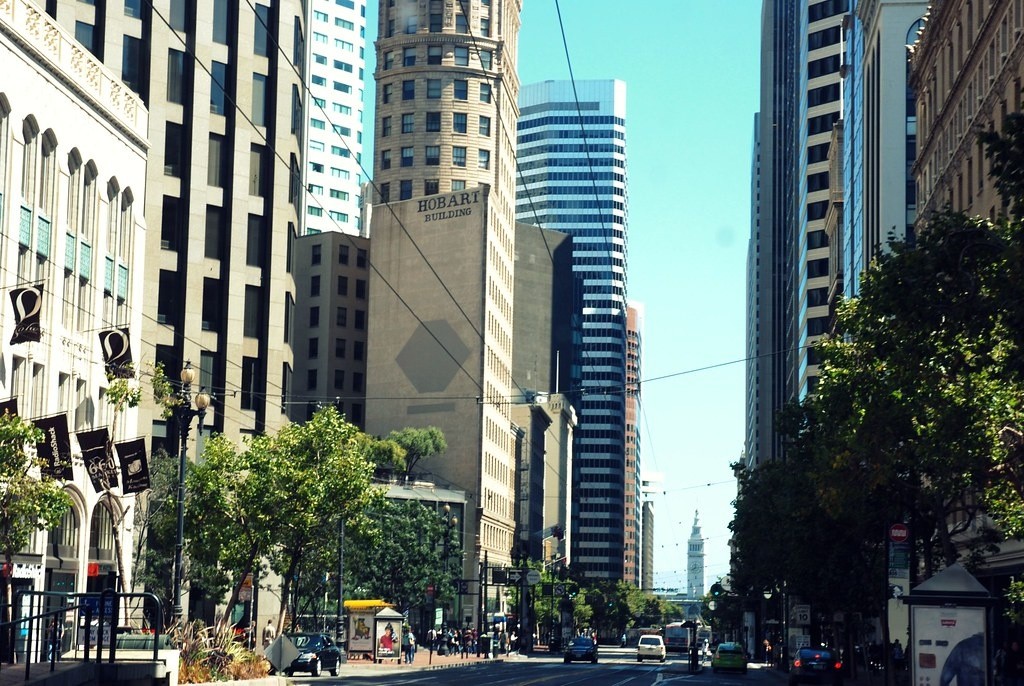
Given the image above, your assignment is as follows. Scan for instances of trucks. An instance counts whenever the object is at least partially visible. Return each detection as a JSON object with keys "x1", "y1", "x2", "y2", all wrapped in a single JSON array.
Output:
[{"x1": 697, "y1": 626, "x2": 712, "y2": 643}]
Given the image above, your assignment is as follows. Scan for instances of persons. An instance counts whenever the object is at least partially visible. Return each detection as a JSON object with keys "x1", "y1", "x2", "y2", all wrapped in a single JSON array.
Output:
[
  {"x1": 592, "y1": 632, "x2": 597, "y2": 645},
  {"x1": 243, "y1": 621, "x2": 255, "y2": 650},
  {"x1": 426, "y1": 626, "x2": 518, "y2": 655},
  {"x1": 380, "y1": 626, "x2": 393, "y2": 652},
  {"x1": 1003, "y1": 641, "x2": 1024, "y2": 686},
  {"x1": 296, "y1": 624, "x2": 302, "y2": 633},
  {"x1": 46, "y1": 616, "x2": 65, "y2": 661},
  {"x1": 401, "y1": 625, "x2": 416, "y2": 664},
  {"x1": 702, "y1": 639, "x2": 709, "y2": 663},
  {"x1": 621, "y1": 634, "x2": 626, "y2": 647},
  {"x1": 262, "y1": 619, "x2": 275, "y2": 649},
  {"x1": 357, "y1": 621, "x2": 371, "y2": 639},
  {"x1": 349, "y1": 653, "x2": 372, "y2": 660},
  {"x1": 869, "y1": 639, "x2": 906, "y2": 676},
  {"x1": 580, "y1": 633, "x2": 584, "y2": 638}
]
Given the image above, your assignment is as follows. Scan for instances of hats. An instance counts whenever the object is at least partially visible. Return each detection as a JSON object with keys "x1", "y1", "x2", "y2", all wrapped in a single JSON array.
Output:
[{"x1": 704, "y1": 639, "x2": 708, "y2": 643}]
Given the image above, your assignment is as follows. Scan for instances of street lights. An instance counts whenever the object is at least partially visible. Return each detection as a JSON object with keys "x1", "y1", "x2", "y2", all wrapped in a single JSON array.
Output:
[
  {"x1": 161, "y1": 358, "x2": 210, "y2": 635},
  {"x1": 443, "y1": 501, "x2": 458, "y2": 573},
  {"x1": 550, "y1": 559, "x2": 561, "y2": 645},
  {"x1": 763, "y1": 583, "x2": 789, "y2": 673}
]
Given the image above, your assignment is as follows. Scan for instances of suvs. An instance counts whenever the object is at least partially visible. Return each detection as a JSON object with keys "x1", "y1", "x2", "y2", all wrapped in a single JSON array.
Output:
[{"x1": 637, "y1": 635, "x2": 666, "y2": 662}]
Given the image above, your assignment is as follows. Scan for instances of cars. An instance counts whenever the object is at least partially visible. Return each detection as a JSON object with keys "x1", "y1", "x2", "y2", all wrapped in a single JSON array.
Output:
[
  {"x1": 286, "y1": 632, "x2": 341, "y2": 677},
  {"x1": 711, "y1": 642, "x2": 750, "y2": 674},
  {"x1": 563, "y1": 638, "x2": 598, "y2": 664},
  {"x1": 788, "y1": 647, "x2": 843, "y2": 684}
]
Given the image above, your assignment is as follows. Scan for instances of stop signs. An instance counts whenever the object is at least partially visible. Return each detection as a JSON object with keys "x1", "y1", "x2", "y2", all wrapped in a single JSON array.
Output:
[{"x1": 426, "y1": 585, "x2": 434, "y2": 597}]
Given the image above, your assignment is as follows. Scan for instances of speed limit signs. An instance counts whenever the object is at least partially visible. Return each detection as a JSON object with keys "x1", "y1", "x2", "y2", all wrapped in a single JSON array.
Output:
[{"x1": 796, "y1": 604, "x2": 811, "y2": 624}]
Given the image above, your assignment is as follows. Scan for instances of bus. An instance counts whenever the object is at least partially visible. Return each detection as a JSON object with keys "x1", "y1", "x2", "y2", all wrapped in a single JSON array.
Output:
[{"x1": 665, "y1": 623, "x2": 690, "y2": 652}]
